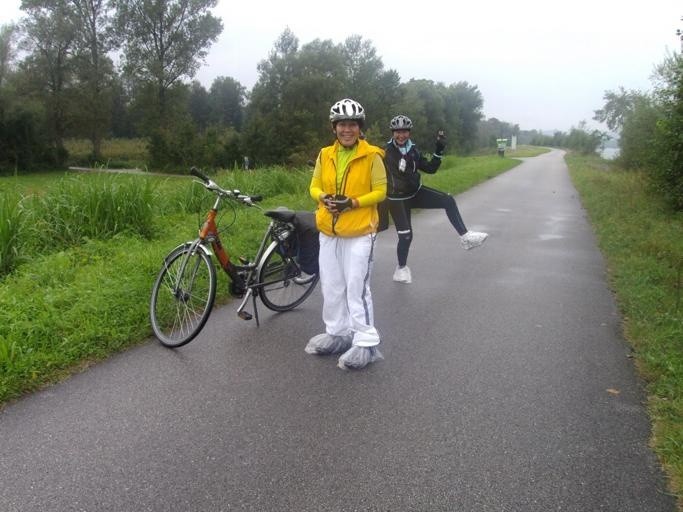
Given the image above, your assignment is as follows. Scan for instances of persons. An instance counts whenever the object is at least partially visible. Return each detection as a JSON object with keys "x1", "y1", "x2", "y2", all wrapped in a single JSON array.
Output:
[
  {"x1": 310, "y1": 98, "x2": 388, "y2": 370},
  {"x1": 383, "y1": 114, "x2": 487, "y2": 283}
]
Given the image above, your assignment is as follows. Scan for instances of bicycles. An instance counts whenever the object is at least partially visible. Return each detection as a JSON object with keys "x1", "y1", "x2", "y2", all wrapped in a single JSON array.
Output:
[{"x1": 145, "y1": 162, "x2": 319, "y2": 353}]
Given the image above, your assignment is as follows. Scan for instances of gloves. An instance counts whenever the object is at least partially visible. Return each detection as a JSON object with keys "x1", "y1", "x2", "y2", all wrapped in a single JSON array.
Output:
[
  {"x1": 433, "y1": 130, "x2": 448, "y2": 155},
  {"x1": 323, "y1": 194, "x2": 353, "y2": 216}
]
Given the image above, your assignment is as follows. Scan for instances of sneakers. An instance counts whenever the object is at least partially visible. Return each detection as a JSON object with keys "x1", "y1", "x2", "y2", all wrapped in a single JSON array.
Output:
[
  {"x1": 307, "y1": 332, "x2": 352, "y2": 354},
  {"x1": 392, "y1": 263, "x2": 411, "y2": 284},
  {"x1": 338, "y1": 344, "x2": 377, "y2": 367},
  {"x1": 461, "y1": 230, "x2": 488, "y2": 249}
]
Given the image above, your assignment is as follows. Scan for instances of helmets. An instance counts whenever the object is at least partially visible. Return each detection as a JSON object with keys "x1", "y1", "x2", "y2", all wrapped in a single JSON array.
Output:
[
  {"x1": 329, "y1": 98, "x2": 366, "y2": 123},
  {"x1": 389, "y1": 114, "x2": 413, "y2": 131}
]
